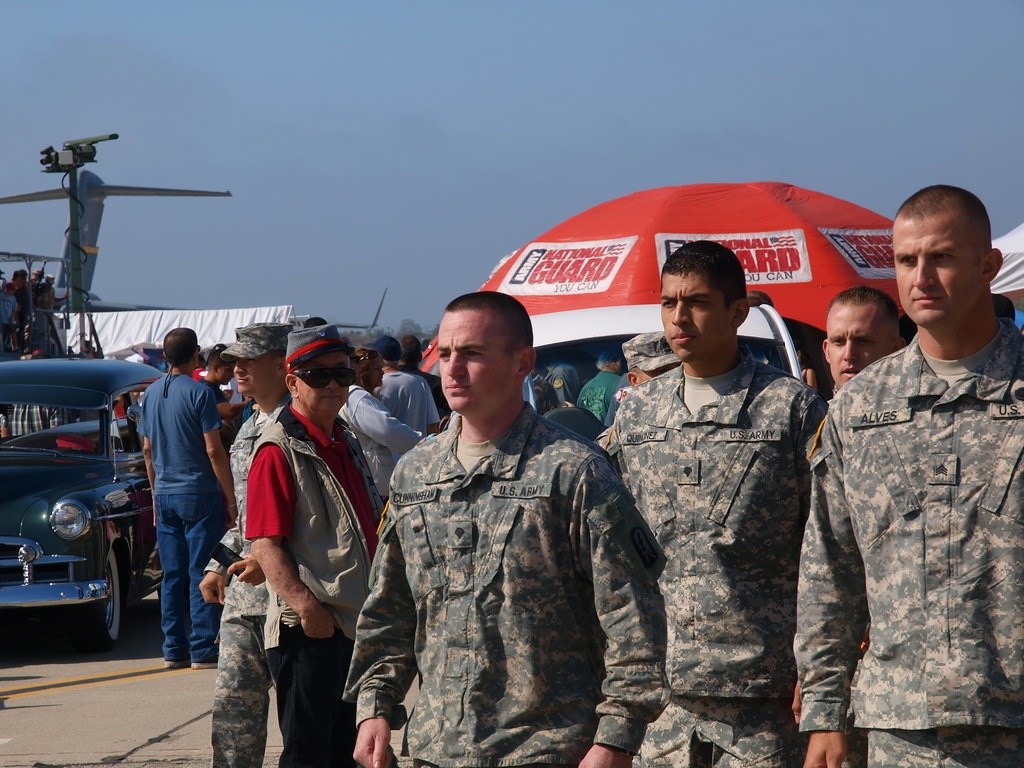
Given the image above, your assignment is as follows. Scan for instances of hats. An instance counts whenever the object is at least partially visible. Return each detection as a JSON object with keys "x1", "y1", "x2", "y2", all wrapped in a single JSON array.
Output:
[
  {"x1": 32, "y1": 268, "x2": 45, "y2": 279},
  {"x1": 46, "y1": 274, "x2": 55, "y2": 285},
  {"x1": 621, "y1": 331, "x2": 682, "y2": 372},
  {"x1": 286, "y1": 323, "x2": 355, "y2": 372},
  {"x1": 220, "y1": 323, "x2": 291, "y2": 360},
  {"x1": 362, "y1": 334, "x2": 403, "y2": 361}
]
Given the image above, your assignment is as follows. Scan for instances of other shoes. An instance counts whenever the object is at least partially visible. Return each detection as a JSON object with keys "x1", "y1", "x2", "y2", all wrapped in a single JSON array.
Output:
[
  {"x1": 190, "y1": 653, "x2": 218, "y2": 669},
  {"x1": 163, "y1": 653, "x2": 191, "y2": 669}
]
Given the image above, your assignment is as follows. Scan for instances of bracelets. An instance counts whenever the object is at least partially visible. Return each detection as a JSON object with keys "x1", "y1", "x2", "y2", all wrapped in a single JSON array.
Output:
[{"x1": 228, "y1": 503, "x2": 236, "y2": 507}]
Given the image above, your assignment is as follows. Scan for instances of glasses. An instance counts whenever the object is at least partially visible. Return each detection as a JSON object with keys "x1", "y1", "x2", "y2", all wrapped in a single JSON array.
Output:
[
  {"x1": 289, "y1": 366, "x2": 358, "y2": 389},
  {"x1": 357, "y1": 350, "x2": 379, "y2": 362}
]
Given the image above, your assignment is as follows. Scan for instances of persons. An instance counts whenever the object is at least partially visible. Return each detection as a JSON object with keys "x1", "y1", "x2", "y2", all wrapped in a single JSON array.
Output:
[
  {"x1": 0, "y1": 269, "x2": 68, "y2": 353},
  {"x1": 136, "y1": 328, "x2": 238, "y2": 671},
  {"x1": 198, "y1": 343, "x2": 252, "y2": 456},
  {"x1": 199, "y1": 323, "x2": 293, "y2": 768},
  {"x1": 245, "y1": 316, "x2": 452, "y2": 768},
  {"x1": 0, "y1": 350, "x2": 68, "y2": 437},
  {"x1": 342, "y1": 239, "x2": 830, "y2": 768},
  {"x1": 791, "y1": 184, "x2": 1024, "y2": 768}
]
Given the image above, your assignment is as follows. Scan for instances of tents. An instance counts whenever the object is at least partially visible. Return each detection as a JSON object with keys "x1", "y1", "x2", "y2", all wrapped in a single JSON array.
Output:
[
  {"x1": 990, "y1": 223, "x2": 1024, "y2": 293},
  {"x1": 53, "y1": 305, "x2": 311, "y2": 360}
]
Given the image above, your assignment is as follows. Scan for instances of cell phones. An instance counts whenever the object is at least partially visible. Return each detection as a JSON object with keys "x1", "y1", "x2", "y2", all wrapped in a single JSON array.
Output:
[{"x1": 209, "y1": 543, "x2": 247, "y2": 577}]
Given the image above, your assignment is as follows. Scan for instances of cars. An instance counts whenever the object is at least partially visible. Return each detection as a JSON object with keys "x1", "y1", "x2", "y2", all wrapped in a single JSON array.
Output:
[
  {"x1": 520, "y1": 299, "x2": 803, "y2": 440},
  {"x1": 0, "y1": 354, "x2": 172, "y2": 656}
]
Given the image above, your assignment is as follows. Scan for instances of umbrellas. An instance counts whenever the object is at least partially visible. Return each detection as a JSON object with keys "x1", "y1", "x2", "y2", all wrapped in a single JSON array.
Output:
[{"x1": 476, "y1": 181, "x2": 904, "y2": 349}]
